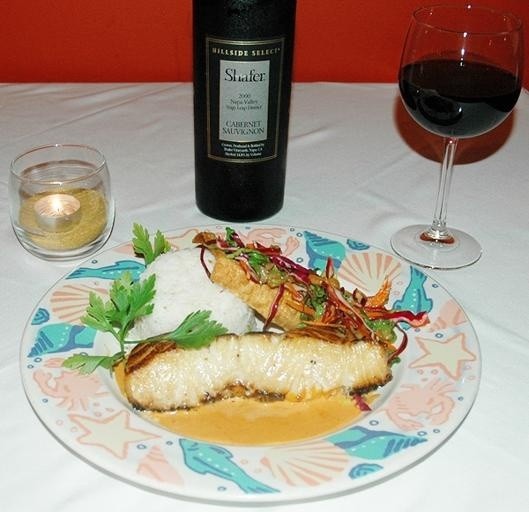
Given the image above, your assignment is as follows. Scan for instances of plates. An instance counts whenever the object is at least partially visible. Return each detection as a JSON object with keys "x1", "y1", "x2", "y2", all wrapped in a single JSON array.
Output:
[{"x1": 19, "y1": 224, "x2": 484, "y2": 508}]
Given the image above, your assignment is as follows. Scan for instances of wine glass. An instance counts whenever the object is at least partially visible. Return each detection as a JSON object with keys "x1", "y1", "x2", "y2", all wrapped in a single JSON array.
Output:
[{"x1": 389, "y1": 4, "x2": 524, "y2": 271}]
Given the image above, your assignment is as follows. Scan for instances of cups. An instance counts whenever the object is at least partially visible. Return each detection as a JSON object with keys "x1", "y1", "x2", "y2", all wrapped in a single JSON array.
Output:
[{"x1": 7, "y1": 143, "x2": 117, "y2": 263}]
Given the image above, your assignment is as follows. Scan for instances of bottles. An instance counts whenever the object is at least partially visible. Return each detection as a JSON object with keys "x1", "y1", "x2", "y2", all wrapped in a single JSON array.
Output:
[{"x1": 192, "y1": 0, "x2": 296, "y2": 223}]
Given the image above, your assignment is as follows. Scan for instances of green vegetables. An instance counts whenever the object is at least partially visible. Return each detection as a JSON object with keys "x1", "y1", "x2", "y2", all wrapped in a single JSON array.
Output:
[
  {"x1": 59, "y1": 269, "x2": 229, "y2": 381},
  {"x1": 130, "y1": 224, "x2": 172, "y2": 268}
]
